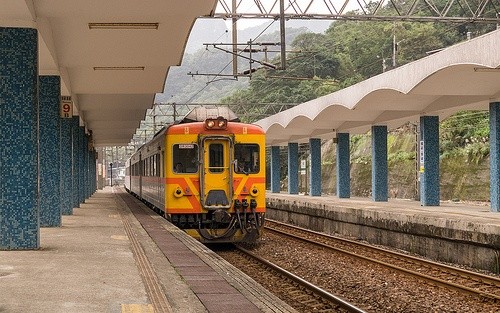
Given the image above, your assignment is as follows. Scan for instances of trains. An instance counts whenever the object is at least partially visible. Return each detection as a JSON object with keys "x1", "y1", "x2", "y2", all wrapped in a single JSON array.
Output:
[{"x1": 123, "y1": 105, "x2": 268, "y2": 251}]
